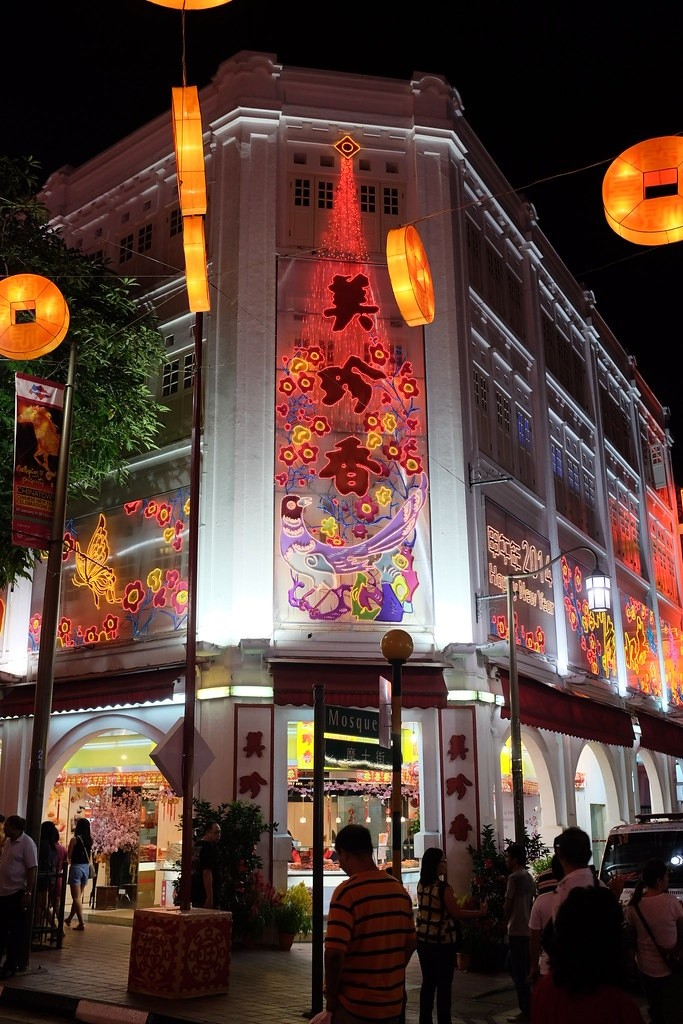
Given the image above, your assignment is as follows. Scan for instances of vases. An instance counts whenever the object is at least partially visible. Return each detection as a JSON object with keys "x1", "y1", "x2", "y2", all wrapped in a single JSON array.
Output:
[{"x1": 238, "y1": 928, "x2": 297, "y2": 952}]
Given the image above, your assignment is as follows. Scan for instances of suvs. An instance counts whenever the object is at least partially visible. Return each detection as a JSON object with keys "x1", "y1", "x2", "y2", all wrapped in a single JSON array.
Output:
[{"x1": 537, "y1": 813, "x2": 683, "y2": 974}]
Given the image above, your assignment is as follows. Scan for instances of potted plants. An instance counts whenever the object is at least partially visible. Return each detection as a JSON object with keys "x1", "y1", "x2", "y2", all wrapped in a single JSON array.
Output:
[{"x1": 455, "y1": 825, "x2": 542, "y2": 975}]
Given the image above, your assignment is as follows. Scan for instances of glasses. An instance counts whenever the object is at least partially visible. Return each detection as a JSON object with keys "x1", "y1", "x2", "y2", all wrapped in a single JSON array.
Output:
[{"x1": 440, "y1": 859, "x2": 448, "y2": 864}]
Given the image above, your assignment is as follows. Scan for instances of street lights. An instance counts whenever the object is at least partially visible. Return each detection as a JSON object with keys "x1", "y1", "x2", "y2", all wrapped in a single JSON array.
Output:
[
  {"x1": 506, "y1": 546, "x2": 615, "y2": 846},
  {"x1": 382, "y1": 631, "x2": 414, "y2": 882}
]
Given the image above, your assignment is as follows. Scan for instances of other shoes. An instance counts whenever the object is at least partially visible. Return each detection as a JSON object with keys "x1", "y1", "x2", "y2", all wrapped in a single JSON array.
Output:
[
  {"x1": 507, "y1": 1013, "x2": 530, "y2": 1023},
  {"x1": 53, "y1": 930, "x2": 65, "y2": 937},
  {"x1": 73, "y1": 924, "x2": 84, "y2": 930},
  {"x1": 65, "y1": 919, "x2": 71, "y2": 926},
  {"x1": 0, "y1": 967, "x2": 16, "y2": 980}
]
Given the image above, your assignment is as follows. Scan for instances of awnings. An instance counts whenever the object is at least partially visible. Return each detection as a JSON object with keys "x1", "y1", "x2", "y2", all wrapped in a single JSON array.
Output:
[
  {"x1": 273, "y1": 662, "x2": 449, "y2": 709},
  {"x1": 634, "y1": 709, "x2": 683, "y2": 759},
  {"x1": 497, "y1": 667, "x2": 636, "y2": 748},
  {"x1": 0, "y1": 665, "x2": 186, "y2": 719}
]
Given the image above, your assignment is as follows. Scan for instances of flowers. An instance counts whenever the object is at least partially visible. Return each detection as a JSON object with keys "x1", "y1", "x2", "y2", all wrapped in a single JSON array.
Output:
[{"x1": 237, "y1": 860, "x2": 313, "y2": 939}]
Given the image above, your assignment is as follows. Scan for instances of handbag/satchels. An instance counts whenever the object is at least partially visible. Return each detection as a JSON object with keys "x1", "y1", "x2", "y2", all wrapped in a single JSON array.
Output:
[
  {"x1": 89, "y1": 865, "x2": 96, "y2": 878},
  {"x1": 659, "y1": 942, "x2": 683, "y2": 976}
]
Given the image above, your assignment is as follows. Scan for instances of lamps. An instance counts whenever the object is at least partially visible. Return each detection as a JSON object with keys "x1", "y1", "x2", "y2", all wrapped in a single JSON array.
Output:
[
  {"x1": 172, "y1": 84, "x2": 208, "y2": 215},
  {"x1": 602, "y1": 135, "x2": 683, "y2": 246},
  {"x1": 385, "y1": 226, "x2": 435, "y2": 328},
  {"x1": 183, "y1": 216, "x2": 212, "y2": 312},
  {"x1": 0, "y1": 273, "x2": 70, "y2": 360}
]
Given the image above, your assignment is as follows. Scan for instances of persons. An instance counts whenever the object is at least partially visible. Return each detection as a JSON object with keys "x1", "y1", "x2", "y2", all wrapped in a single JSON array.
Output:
[
  {"x1": 287, "y1": 830, "x2": 301, "y2": 866},
  {"x1": 503, "y1": 826, "x2": 683, "y2": 1024},
  {"x1": 310, "y1": 824, "x2": 416, "y2": 1024},
  {"x1": 323, "y1": 830, "x2": 340, "y2": 864},
  {"x1": 0, "y1": 813, "x2": 93, "y2": 978},
  {"x1": 416, "y1": 847, "x2": 488, "y2": 1024},
  {"x1": 192, "y1": 820, "x2": 223, "y2": 908}
]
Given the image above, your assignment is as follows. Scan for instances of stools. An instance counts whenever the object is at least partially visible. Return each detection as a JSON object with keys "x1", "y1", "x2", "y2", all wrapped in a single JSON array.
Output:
[{"x1": 95, "y1": 886, "x2": 119, "y2": 909}]
